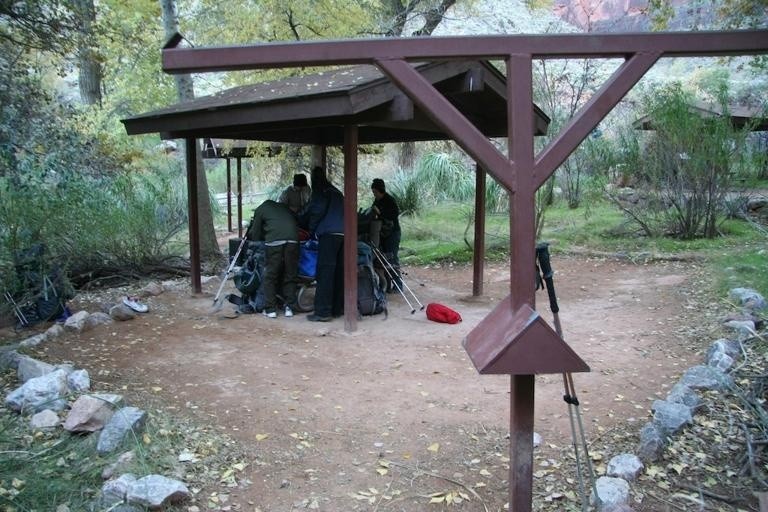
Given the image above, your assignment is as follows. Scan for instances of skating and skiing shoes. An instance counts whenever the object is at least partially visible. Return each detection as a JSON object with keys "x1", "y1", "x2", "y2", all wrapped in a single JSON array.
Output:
[{"x1": 122, "y1": 295, "x2": 149, "y2": 313}]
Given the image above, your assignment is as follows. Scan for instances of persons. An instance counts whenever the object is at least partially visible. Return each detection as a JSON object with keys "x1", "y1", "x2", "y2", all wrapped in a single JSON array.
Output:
[
  {"x1": 370, "y1": 179, "x2": 401, "y2": 293},
  {"x1": 254, "y1": 200, "x2": 299, "y2": 318},
  {"x1": 279, "y1": 174, "x2": 311, "y2": 216},
  {"x1": 307, "y1": 166, "x2": 344, "y2": 322}
]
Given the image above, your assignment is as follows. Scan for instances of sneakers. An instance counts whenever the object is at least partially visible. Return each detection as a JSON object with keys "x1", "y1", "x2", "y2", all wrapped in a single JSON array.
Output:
[
  {"x1": 262, "y1": 309, "x2": 278, "y2": 318},
  {"x1": 370, "y1": 178, "x2": 385, "y2": 193},
  {"x1": 387, "y1": 288, "x2": 403, "y2": 294},
  {"x1": 284, "y1": 305, "x2": 294, "y2": 317},
  {"x1": 306, "y1": 314, "x2": 333, "y2": 322}
]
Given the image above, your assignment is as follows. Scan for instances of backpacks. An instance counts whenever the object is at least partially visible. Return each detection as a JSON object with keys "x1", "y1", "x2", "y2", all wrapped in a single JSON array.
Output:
[
  {"x1": 425, "y1": 302, "x2": 463, "y2": 325},
  {"x1": 357, "y1": 239, "x2": 387, "y2": 315}
]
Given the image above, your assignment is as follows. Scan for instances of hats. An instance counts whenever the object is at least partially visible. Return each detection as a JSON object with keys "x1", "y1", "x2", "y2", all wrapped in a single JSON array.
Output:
[{"x1": 293, "y1": 174, "x2": 307, "y2": 187}]
